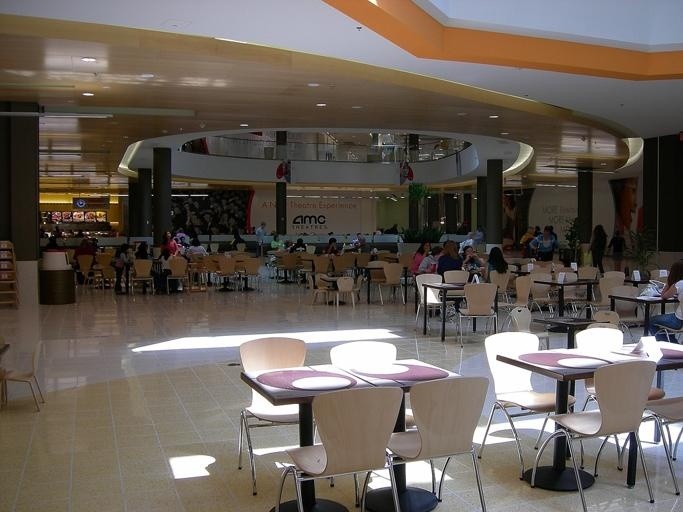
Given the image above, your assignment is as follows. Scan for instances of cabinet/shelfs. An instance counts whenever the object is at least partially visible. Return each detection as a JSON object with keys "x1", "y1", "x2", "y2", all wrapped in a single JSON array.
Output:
[{"x1": 0, "y1": 240, "x2": 19, "y2": 310}]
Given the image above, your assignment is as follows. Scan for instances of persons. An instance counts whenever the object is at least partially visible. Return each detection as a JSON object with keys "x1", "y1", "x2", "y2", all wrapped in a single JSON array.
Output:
[
  {"x1": 607, "y1": 230, "x2": 626, "y2": 270},
  {"x1": 521, "y1": 225, "x2": 558, "y2": 262},
  {"x1": 648, "y1": 262, "x2": 683, "y2": 343},
  {"x1": 256, "y1": 223, "x2": 266, "y2": 257},
  {"x1": 412, "y1": 240, "x2": 508, "y2": 285},
  {"x1": 73, "y1": 238, "x2": 97, "y2": 285},
  {"x1": 115, "y1": 228, "x2": 206, "y2": 294},
  {"x1": 40, "y1": 225, "x2": 62, "y2": 250},
  {"x1": 387, "y1": 224, "x2": 398, "y2": 235},
  {"x1": 269, "y1": 234, "x2": 370, "y2": 257},
  {"x1": 587, "y1": 225, "x2": 608, "y2": 278},
  {"x1": 230, "y1": 231, "x2": 246, "y2": 250}
]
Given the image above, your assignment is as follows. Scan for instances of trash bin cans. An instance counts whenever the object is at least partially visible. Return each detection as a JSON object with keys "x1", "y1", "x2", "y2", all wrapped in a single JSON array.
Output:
[{"x1": 39, "y1": 249, "x2": 76, "y2": 305}]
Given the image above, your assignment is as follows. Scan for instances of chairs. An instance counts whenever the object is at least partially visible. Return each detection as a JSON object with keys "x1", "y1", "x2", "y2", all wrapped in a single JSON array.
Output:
[
  {"x1": 478, "y1": 331, "x2": 586, "y2": 480},
  {"x1": 238, "y1": 337, "x2": 336, "y2": 495},
  {"x1": 361, "y1": 376, "x2": 490, "y2": 511},
  {"x1": 413, "y1": 270, "x2": 533, "y2": 347},
  {"x1": 530, "y1": 360, "x2": 657, "y2": 512},
  {"x1": 329, "y1": 340, "x2": 437, "y2": 508},
  {"x1": 535, "y1": 285, "x2": 683, "y2": 370},
  {"x1": 67, "y1": 245, "x2": 683, "y2": 318},
  {"x1": 576, "y1": 326, "x2": 673, "y2": 469},
  {"x1": 0, "y1": 340, "x2": 45, "y2": 412}
]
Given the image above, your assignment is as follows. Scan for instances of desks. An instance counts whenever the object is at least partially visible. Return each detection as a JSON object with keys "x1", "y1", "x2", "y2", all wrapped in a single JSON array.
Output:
[
  {"x1": 240, "y1": 359, "x2": 461, "y2": 512},
  {"x1": 495, "y1": 341, "x2": 683, "y2": 496}
]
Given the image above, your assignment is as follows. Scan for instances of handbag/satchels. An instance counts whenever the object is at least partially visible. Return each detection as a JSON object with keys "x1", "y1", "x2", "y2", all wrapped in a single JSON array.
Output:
[{"x1": 640, "y1": 279, "x2": 666, "y2": 296}]
